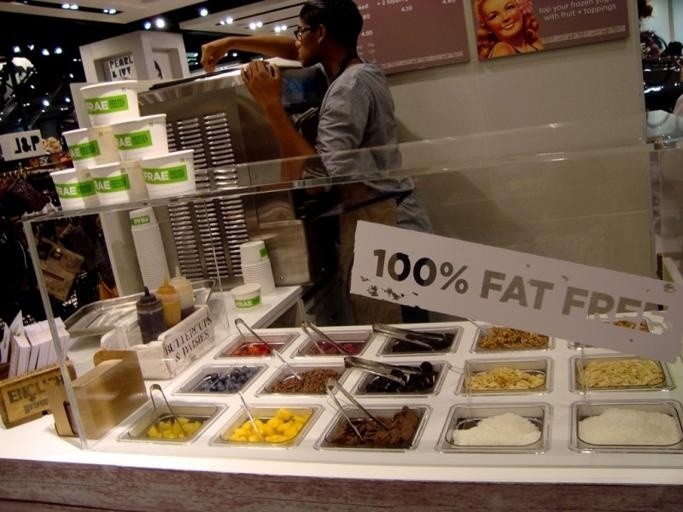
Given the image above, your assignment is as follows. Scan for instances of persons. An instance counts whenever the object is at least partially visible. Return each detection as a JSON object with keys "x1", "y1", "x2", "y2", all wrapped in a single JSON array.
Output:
[
  {"x1": 474, "y1": 1, "x2": 545, "y2": 59},
  {"x1": 200, "y1": 0, "x2": 431, "y2": 324}
]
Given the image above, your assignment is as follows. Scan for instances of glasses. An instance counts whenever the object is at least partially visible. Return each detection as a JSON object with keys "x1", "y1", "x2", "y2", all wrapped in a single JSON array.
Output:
[{"x1": 292, "y1": 26, "x2": 311, "y2": 42}]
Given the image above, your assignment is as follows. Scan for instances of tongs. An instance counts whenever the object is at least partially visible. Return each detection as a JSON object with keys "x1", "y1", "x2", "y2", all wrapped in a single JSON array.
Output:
[{"x1": 147, "y1": 318, "x2": 447, "y2": 446}]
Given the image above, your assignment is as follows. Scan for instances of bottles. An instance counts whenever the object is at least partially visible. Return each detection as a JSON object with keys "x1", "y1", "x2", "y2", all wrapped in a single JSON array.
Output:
[{"x1": 134, "y1": 266, "x2": 194, "y2": 346}]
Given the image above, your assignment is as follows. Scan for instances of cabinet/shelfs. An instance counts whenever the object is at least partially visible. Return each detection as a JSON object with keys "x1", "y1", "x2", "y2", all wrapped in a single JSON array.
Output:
[{"x1": 0, "y1": 110, "x2": 683, "y2": 512}]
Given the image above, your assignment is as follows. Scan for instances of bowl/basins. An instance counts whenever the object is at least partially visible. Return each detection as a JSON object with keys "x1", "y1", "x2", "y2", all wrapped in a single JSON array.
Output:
[
  {"x1": 48, "y1": 80, "x2": 198, "y2": 210},
  {"x1": 127, "y1": 209, "x2": 173, "y2": 292},
  {"x1": 231, "y1": 241, "x2": 276, "y2": 314}
]
